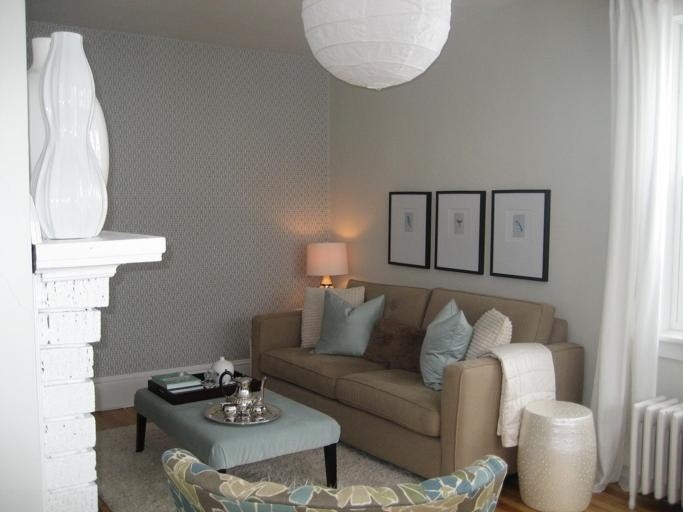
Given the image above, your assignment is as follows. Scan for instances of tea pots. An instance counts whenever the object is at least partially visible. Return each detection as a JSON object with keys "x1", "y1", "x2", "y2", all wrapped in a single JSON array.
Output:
[{"x1": 219, "y1": 369, "x2": 268, "y2": 415}]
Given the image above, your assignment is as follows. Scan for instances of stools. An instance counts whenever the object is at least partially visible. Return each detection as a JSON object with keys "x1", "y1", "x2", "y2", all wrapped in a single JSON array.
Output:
[{"x1": 517, "y1": 400, "x2": 598, "y2": 511}]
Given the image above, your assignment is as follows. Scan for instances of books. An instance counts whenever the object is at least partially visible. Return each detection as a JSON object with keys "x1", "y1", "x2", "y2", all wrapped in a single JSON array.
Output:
[{"x1": 151, "y1": 370, "x2": 204, "y2": 395}]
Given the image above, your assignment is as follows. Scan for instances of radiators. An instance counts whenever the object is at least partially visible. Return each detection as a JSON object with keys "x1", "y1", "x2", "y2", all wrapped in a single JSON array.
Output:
[{"x1": 628, "y1": 396, "x2": 683, "y2": 511}]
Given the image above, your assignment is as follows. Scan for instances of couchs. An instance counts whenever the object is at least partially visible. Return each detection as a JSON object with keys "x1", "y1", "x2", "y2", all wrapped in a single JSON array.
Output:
[{"x1": 251, "y1": 279, "x2": 585, "y2": 479}]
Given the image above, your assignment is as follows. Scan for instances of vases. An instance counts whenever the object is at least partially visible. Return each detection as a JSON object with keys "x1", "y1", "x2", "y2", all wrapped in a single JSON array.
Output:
[{"x1": 28, "y1": 31, "x2": 110, "y2": 240}]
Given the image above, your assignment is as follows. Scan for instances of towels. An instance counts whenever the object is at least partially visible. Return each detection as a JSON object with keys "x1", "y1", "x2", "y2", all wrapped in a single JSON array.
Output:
[{"x1": 476, "y1": 342, "x2": 556, "y2": 449}]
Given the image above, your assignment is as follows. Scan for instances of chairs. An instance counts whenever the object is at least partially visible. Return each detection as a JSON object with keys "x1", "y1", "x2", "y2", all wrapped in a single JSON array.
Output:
[{"x1": 163, "y1": 448, "x2": 509, "y2": 511}]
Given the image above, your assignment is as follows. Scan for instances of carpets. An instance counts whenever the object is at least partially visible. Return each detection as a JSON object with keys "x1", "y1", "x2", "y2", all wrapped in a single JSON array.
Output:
[{"x1": 98, "y1": 423, "x2": 426, "y2": 512}]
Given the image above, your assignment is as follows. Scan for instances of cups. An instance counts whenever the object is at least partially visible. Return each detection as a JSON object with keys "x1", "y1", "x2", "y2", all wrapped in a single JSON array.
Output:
[
  {"x1": 223, "y1": 403, "x2": 239, "y2": 419},
  {"x1": 249, "y1": 400, "x2": 267, "y2": 421},
  {"x1": 204, "y1": 370, "x2": 217, "y2": 389}
]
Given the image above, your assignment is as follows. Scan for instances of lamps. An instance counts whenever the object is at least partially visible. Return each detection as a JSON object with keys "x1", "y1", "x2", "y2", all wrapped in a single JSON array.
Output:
[
  {"x1": 307, "y1": 242, "x2": 348, "y2": 288},
  {"x1": 301, "y1": 0, "x2": 452, "y2": 90}
]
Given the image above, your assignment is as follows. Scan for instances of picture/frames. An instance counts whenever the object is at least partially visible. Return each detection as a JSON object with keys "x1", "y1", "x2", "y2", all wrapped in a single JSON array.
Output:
[
  {"x1": 388, "y1": 191, "x2": 431, "y2": 269},
  {"x1": 490, "y1": 189, "x2": 551, "y2": 281},
  {"x1": 435, "y1": 191, "x2": 486, "y2": 274}
]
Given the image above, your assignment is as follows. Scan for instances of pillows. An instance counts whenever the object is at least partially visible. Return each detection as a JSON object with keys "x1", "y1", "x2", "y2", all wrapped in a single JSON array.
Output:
[
  {"x1": 419, "y1": 298, "x2": 473, "y2": 391},
  {"x1": 465, "y1": 308, "x2": 512, "y2": 361},
  {"x1": 300, "y1": 286, "x2": 365, "y2": 349},
  {"x1": 362, "y1": 317, "x2": 424, "y2": 373},
  {"x1": 309, "y1": 290, "x2": 385, "y2": 356}
]
